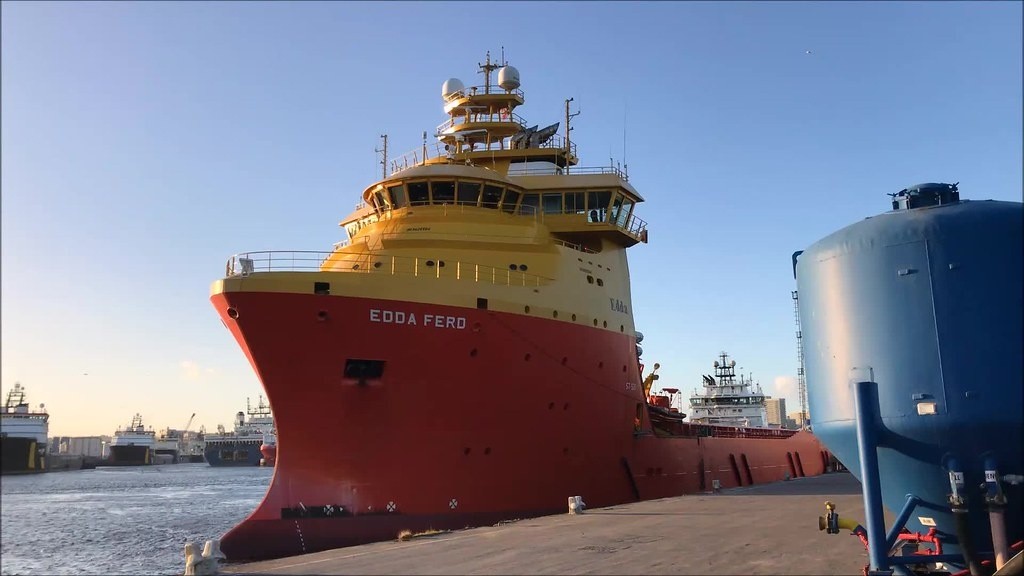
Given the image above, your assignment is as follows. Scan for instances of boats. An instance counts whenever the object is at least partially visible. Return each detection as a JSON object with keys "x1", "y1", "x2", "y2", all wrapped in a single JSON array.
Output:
[
  {"x1": 108, "y1": 413, "x2": 173, "y2": 466},
  {"x1": 1, "y1": 381, "x2": 84, "y2": 475},
  {"x1": 209, "y1": 45, "x2": 832, "y2": 562},
  {"x1": 205, "y1": 394, "x2": 276, "y2": 466},
  {"x1": 154, "y1": 427, "x2": 205, "y2": 464}
]
